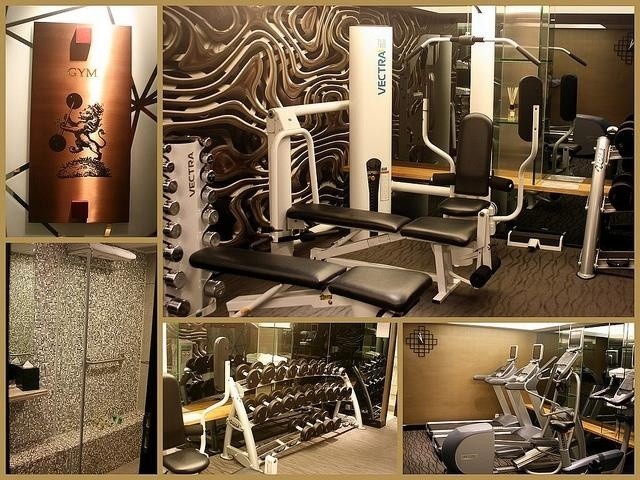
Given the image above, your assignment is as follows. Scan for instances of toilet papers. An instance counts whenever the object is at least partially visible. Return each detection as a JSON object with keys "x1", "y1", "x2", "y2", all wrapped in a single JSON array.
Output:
[{"x1": 8, "y1": 357, "x2": 24, "y2": 386}]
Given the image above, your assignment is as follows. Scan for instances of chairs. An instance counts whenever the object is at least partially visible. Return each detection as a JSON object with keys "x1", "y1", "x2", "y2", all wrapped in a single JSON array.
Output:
[
  {"x1": 163, "y1": 373, "x2": 210, "y2": 474},
  {"x1": 430, "y1": 113, "x2": 514, "y2": 218}
]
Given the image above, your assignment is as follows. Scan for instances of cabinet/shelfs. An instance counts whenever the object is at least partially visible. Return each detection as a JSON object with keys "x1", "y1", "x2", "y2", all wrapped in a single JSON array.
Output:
[
  {"x1": 220, "y1": 367, "x2": 367, "y2": 475},
  {"x1": 352, "y1": 365, "x2": 387, "y2": 428}
]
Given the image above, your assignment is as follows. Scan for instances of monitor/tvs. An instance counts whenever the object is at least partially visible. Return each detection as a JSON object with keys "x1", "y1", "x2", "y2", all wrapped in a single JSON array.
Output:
[
  {"x1": 532, "y1": 343, "x2": 544, "y2": 359},
  {"x1": 605, "y1": 350, "x2": 618, "y2": 364},
  {"x1": 509, "y1": 345, "x2": 518, "y2": 359},
  {"x1": 567, "y1": 327, "x2": 583, "y2": 348}
]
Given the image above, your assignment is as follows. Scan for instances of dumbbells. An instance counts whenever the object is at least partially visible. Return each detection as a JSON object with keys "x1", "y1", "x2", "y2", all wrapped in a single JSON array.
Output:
[
  {"x1": 237, "y1": 359, "x2": 352, "y2": 442},
  {"x1": 608, "y1": 172, "x2": 634, "y2": 210},
  {"x1": 614, "y1": 121, "x2": 634, "y2": 156},
  {"x1": 361, "y1": 356, "x2": 385, "y2": 392}
]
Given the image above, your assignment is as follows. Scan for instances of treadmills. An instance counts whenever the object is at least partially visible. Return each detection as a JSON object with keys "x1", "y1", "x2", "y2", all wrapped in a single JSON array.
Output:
[
  {"x1": 430, "y1": 343, "x2": 544, "y2": 442},
  {"x1": 426, "y1": 344, "x2": 518, "y2": 434}
]
